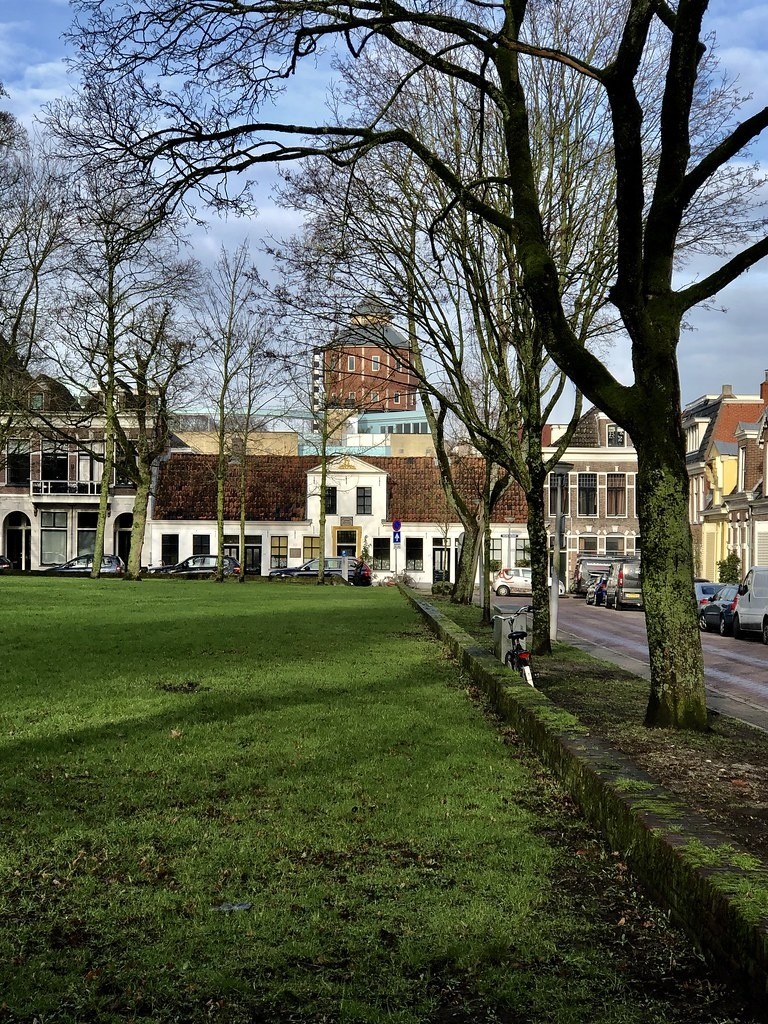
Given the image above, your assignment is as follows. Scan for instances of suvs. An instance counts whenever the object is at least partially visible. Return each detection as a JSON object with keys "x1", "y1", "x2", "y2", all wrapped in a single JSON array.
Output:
[
  {"x1": 570, "y1": 556, "x2": 645, "y2": 610},
  {"x1": 45, "y1": 553, "x2": 124, "y2": 572},
  {"x1": 159, "y1": 554, "x2": 248, "y2": 575},
  {"x1": 269, "y1": 556, "x2": 372, "y2": 588}
]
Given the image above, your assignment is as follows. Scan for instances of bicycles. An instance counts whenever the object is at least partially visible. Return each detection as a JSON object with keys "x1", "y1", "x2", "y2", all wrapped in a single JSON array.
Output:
[
  {"x1": 488, "y1": 606, "x2": 543, "y2": 687},
  {"x1": 383, "y1": 568, "x2": 417, "y2": 589}
]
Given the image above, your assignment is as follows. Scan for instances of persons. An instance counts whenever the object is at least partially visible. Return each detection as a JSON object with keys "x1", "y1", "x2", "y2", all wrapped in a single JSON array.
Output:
[{"x1": 350, "y1": 556, "x2": 364, "y2": 585}]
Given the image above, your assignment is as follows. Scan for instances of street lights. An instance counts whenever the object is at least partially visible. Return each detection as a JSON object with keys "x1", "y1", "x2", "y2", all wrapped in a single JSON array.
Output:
[
  {"x1": 550, "y1": 460, "x2": 574, "y2": 600},
  {"x1": 504, "y1": 517, "x2": 516, "y2": 565}
]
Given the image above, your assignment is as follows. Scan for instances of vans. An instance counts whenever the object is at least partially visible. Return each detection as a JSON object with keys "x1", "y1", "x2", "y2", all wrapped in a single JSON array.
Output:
[{"x1": 492, "y1": 567, "x2": 565, "y2": 597}]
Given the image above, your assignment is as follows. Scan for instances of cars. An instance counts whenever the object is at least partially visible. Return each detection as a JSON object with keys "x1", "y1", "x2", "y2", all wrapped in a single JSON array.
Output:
[
  {"x1": 42, "y1": 551, "x2": 66, "y2": 567},
  {"x1": 246, "y1": 563, "x2": 261, "y2": 575},
  {"x1": 435, "y1": 570, "x2": 448, "y2": 582},
  {"x1": 149, "y1": 565, "x2": 185, "y2": 576},
  {"x1": 692, "y1": 565, "x2": 768, "y2": 644},
  {"x1": 0, "y1": 555, "x2": 14, "y2": 575}
]
